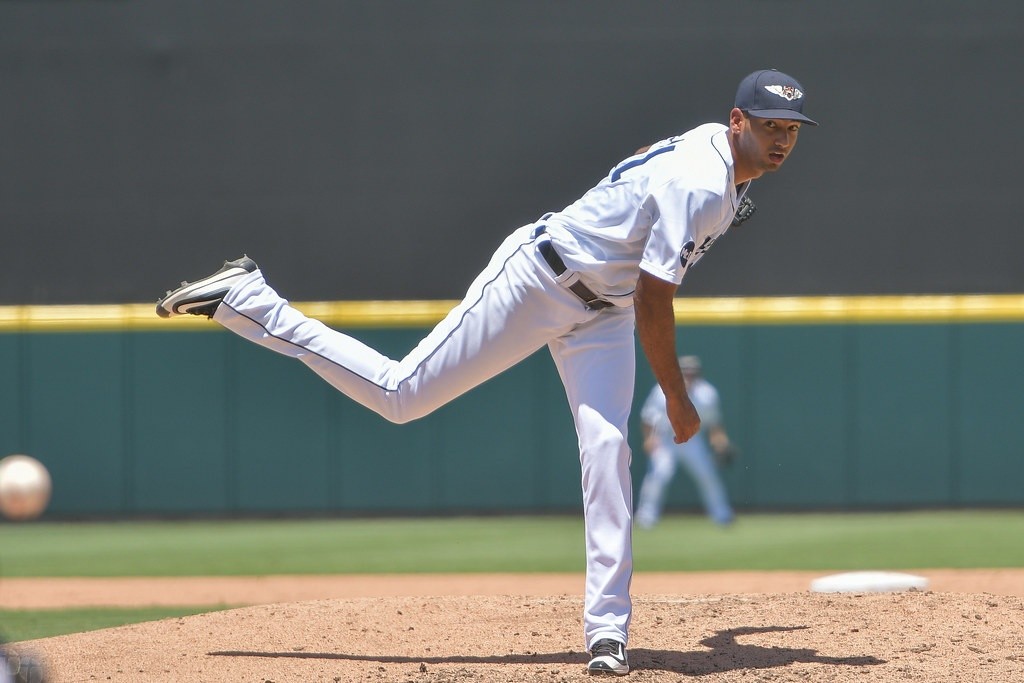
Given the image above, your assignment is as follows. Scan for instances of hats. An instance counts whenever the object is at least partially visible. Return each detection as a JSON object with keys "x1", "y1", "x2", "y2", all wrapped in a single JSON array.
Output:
[{"x1": 733, "y1": 70, "x2": 819, "y2": 127}]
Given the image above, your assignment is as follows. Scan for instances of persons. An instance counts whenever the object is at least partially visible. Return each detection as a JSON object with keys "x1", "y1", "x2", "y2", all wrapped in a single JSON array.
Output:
[
  {"x1": 638, "y1": 353, "x2": 735, "y2": 527},
  {"x1": 154, "y1": 68, "x2": 821, "y2": 678}
]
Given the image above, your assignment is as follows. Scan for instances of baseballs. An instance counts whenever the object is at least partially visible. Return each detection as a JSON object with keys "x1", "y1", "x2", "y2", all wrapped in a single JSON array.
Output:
[{"x1": 0, "y1": 452, "x2": 54, "y2": 518}]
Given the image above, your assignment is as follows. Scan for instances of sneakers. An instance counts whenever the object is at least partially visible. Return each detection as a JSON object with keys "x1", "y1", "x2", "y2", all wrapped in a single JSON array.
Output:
[
  {"x1": 587, "y1": 637, "x2": 632, "y2": 677},
  {"x1": 155, "y1": 256, "x2": 257, "y2": 319}
]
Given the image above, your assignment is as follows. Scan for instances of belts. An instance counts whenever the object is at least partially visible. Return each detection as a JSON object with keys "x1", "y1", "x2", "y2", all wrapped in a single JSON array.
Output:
[{"x1": 537, "y1": 213, "x2": 613, "y2": 309}]
